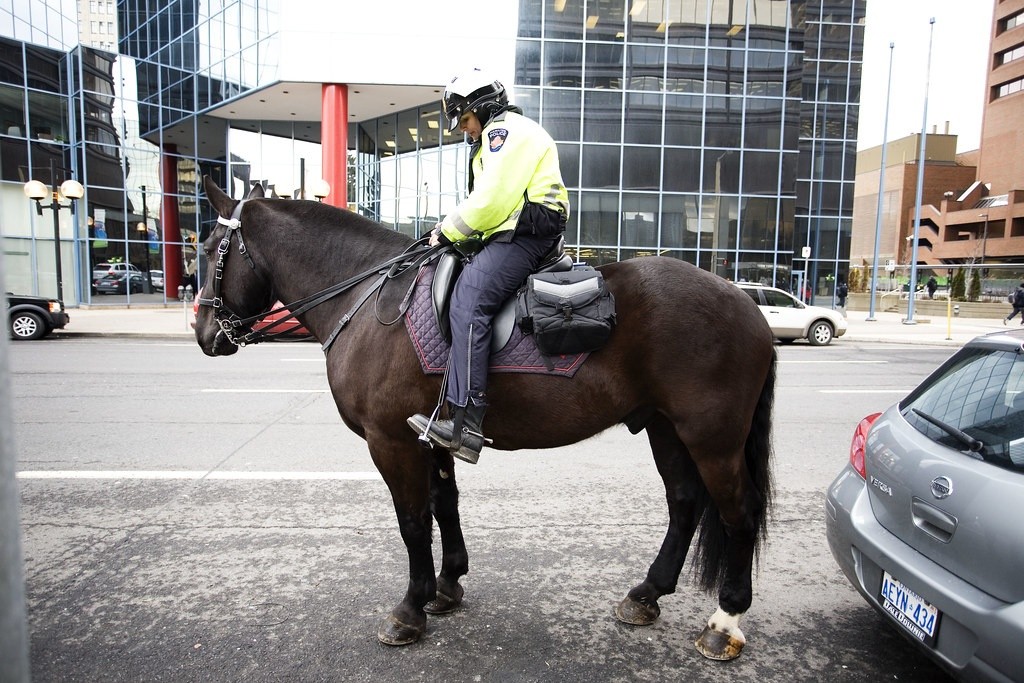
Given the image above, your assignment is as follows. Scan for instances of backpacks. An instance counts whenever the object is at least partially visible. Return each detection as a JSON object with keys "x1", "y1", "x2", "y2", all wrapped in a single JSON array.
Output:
[
  {"x1": 1008, "y1": 292, "x2": 1016, "y2": 304},
  {"x1": 930, "y1": 280, "x2": 937, "y2": 290}
]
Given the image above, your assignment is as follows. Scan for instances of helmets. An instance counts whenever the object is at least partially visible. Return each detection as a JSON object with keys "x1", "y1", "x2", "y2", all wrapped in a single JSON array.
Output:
[{"x1": 443, "y1": 71, "x2": 508, "y2": 133}]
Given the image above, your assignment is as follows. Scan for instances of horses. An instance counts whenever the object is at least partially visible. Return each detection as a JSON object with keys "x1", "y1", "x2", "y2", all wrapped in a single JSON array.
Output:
[{"x1": 192, "y1": 176, "x2": 780, "y2": 661}]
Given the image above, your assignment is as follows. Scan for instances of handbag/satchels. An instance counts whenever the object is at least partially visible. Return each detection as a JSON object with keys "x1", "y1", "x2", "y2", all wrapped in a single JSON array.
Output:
[{"x1": 516, "y1": 269, "x2": 616, "y2": 371}]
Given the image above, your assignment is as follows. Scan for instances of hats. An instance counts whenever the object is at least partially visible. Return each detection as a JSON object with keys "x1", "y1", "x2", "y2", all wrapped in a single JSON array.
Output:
[{"x1": 929, "y1": 276, "x2": 934, "y2": 279}]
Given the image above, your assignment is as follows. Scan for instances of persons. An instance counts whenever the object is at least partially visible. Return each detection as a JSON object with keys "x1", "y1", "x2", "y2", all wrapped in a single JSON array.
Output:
[
  {"x1": 801, "y1": 276, "x2": 812, "y2": 306},
  {"x1": 927, "y1": 276, "x2": 937, "y2": 297},
  {"x1": 1003, "y1": 283, "x2": 1024, "y2": 325},
  {"x1": 406, "y1": 69, "x2": 570, "y2": 465},
  {"x1": 835, "y1": 281, "x2": 847, "y2": 308}
]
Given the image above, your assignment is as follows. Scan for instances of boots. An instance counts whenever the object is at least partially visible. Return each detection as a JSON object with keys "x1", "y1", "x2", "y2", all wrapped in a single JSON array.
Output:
[{"x1": 407, "y1": 389, "x2": 490, "y2": 465}]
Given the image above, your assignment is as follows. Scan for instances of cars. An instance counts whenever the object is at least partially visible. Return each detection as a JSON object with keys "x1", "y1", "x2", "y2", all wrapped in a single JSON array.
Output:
[
  {"x1": 95, "y1": 272, "x2": 143, "y2": 294},
  {"x1": 4, "y1": 293, "x2": 69, "y2": 340},
  {"x1": 826, "y1": 329, "x2": 1024, "y2": 683},
  {"x1": 149, "y1": 270, "x2": 164, "y2": 290}
]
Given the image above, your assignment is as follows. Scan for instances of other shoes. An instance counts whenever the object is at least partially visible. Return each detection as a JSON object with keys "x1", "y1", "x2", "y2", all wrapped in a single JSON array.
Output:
[
  {"x1": 1020, "y1": 322, "x2": 1024, "y2": 325},
  {"x1": 1004, "y1": 317, "x2": 1007, "y2": 325}
]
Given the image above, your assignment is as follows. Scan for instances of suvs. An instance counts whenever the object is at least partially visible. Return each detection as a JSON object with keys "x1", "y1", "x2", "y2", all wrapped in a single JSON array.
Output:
[
  {"x1": 92, "y1": 263, "x2": 142, "y2": 280},
  {"x1": 727, "y1": 281, "x2": 847, "y2": 345}
]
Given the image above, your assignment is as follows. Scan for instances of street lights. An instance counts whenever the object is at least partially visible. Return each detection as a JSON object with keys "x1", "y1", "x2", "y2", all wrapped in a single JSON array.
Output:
[
  {"x1": 138, "y1": 222, "x2": 152, "y2": 293},
  {"x1": 24, "y1": 179, "x2": 84, "y2": 311}
]
